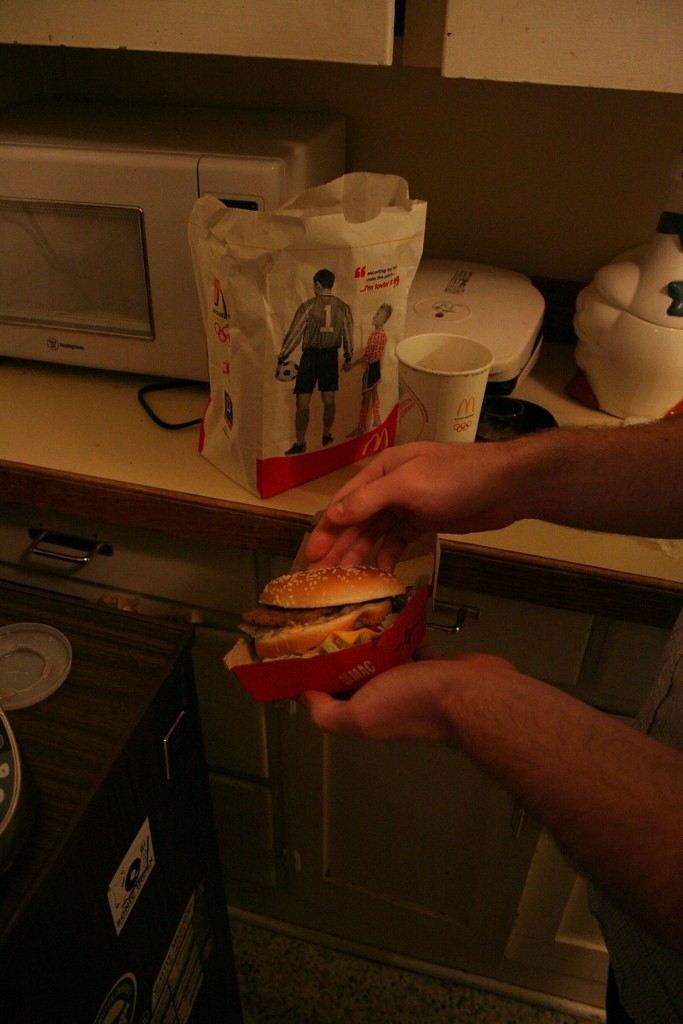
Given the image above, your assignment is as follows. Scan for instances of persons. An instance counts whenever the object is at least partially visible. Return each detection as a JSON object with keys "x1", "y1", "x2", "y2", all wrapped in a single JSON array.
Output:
[{"x1": 300, "y1": 412, "x2": 683, "y2": 1024}]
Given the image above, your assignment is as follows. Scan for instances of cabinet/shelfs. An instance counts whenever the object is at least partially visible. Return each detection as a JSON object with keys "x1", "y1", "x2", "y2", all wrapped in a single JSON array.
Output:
[
  {"x1": 0, "y1": 0, "x2": 683, "y2": 94},
  {"x1": 0, "y1": 505, "x2": 683, "y2": 1024}
]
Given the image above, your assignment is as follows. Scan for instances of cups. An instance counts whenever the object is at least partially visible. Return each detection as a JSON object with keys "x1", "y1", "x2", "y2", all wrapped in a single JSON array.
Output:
[{"x1": 396, "y1": 333, "x2": 494, "y2": 445}]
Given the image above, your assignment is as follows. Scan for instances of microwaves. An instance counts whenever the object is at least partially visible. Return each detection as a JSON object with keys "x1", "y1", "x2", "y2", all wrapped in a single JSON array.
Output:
[{"x1": 0, "y1": 100, "x2": 349, "y2": 383}]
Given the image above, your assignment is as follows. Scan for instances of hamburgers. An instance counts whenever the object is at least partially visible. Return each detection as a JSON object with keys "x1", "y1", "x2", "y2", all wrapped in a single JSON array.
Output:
[{"x1": 234, "y1": 564, "x2": 405, "y2": 661}]
